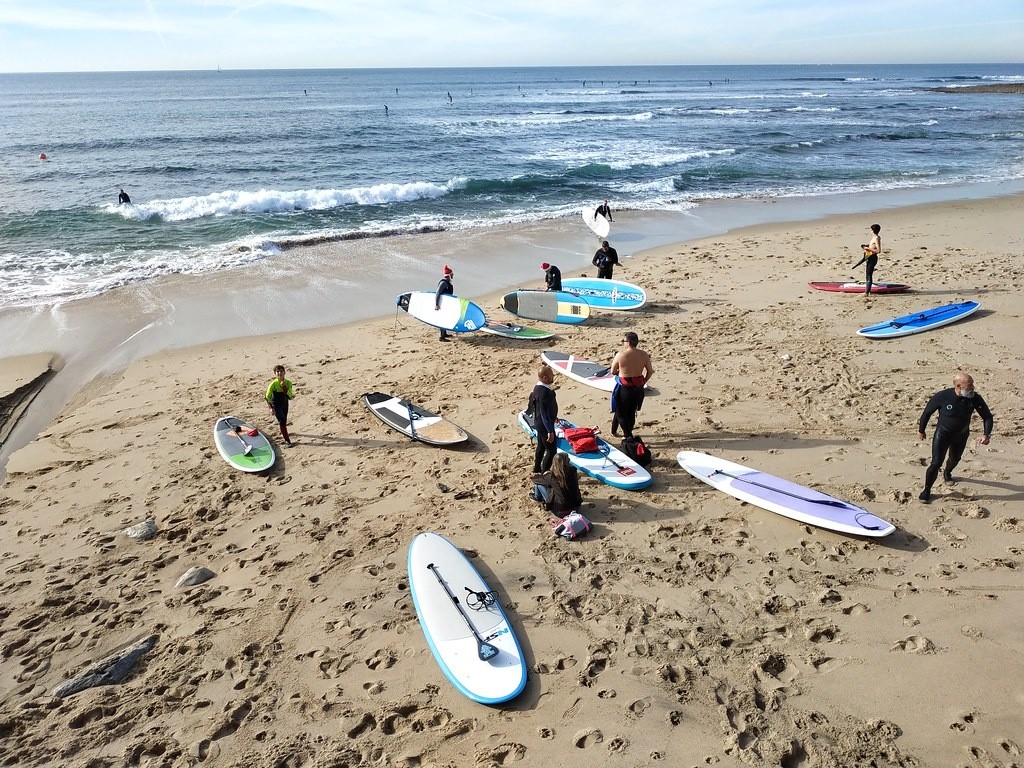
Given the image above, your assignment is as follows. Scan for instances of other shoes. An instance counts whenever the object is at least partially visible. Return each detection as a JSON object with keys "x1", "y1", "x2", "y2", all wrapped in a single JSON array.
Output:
[
  {"x1": 445, "y1": 334, "x2": 452, "y2": 337},
  {"x1": 944, "y1": 470, "x2": 954, "y2": 486},
  {"x1": 439, "y1": 337, "x2": 450, "y2": 342},
  {"x1": 285, "y1": 440, "x2": 292, "y2": 448},
  {"x1": 529, "y1": 493, "x2": 538, "y2": 500},
  {"x1": 919, "y1": 488, "x2": 930, "y2": 503}
]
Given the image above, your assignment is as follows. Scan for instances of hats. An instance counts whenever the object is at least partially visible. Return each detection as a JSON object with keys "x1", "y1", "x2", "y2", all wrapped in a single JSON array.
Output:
[
  {"x1": 540, "y1": 263, "x2": 551, "y2": 272},
  {"x1": 444, "y1": 265, "x2": 452, "y2": 276},
  {"x1": 602, "y1": 241, "x2": 608, "y2": 247}
]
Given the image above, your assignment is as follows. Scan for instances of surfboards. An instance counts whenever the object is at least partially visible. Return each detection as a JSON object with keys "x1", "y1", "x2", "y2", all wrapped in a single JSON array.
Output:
[
  {"x1": 581, "y1": 208, "x2": 611, "y2": 238},
  {"x1": 541, "y1": 348, "x2": 649, "y2": 393},
  {"x1": 677, "y1": 449, "x2": 896, "y2": 538},
  {"x1": 395, "y1": 277, "x2": 646, "y2": 339},
  {"x1": 408, "y1": 532, "x2": 527, "y2": 705},
  {"x1": 365, "y1": 391, "x2": 469, "y2": 446},
  {"x1": 517, "y1": 408, "x2": 655, "y2": 491},
  {"x1": 808, "y1": 281, "x2": 911, "y2": 292},
  {"x1": 214, "y1": 415, "x2": 276, "y2": 472},
  {"x1": 855, "y1": 301, "x2": 982, "y2": 338}
]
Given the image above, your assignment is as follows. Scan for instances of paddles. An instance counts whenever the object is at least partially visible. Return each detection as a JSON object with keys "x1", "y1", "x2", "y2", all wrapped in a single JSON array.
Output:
[
  {"x1": 222, "y1": 418, "x2": 254, "y2": 457},
  {"x1": 428, "y1": 563, "x2": 500, "y2": 662},
  {"x1": 889, "y1": 307, "x2": 957, "y2": 328},
  {"x1": 592, "y1": 367, "x2": 611, "y2": 378},
  {"x1": 558, "y1": 419, "x2": 624, "y2": 470},
  {"x1": 402, "y1": 400, "x2": 420, "y2": 443},
  {"x1": 715, "y1": 469, "x2": 847, "y2": 507}
]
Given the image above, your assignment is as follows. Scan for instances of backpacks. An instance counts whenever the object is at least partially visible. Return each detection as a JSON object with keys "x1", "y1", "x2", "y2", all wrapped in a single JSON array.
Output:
[{"x1": 622, "y1": 435, "x2": 652, "y2": 467}]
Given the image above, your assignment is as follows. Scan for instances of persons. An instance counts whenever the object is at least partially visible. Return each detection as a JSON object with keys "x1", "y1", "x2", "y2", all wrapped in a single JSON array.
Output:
[
  {"x1": 435, "y1": 264, "x2": 453, "y2": 342},
  {"x1": 533, "y1": 366, "x2": 558, "y2": 475},
  {"x1": 118, "y1": 188, "x2": 131, "y2": 204},
  {"x1": 918, "y1": 373, "x2": 994, "y2": 503},
  {"x1": 384, "y1": 105, "x2": 388, "y2": 115},
  {"x1": 594, "y1": 200, "x2": 612, "y2": 240},
  {"x1": 266, "y1": 365, "x2": 292, "y2": 448},
  {"x1": 593, "y1": 241, "x2": 619, "y2": 279},
  {"x1": 450, "y1": 96, "x2": 452, "y2": 106},
  {"x1": 864, "y1": 224, "x2": 881, "y2": 296},
  {"x1": 529, "y1": 452, "x2": 583, "y2": 519},
  {"x1": 611, "y1": 332, "x2": 653, "y2": 439},
  {"x1": 541, "y1": 262, "x2": 562, "y2": 291},
  {"x1": 611, "y1": 376, "x2": 623, "y2": 438}
]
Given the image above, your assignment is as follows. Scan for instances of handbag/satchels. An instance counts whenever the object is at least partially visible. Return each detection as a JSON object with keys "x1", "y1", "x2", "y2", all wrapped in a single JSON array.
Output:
[{"x1": 555, "y1": 510, "x2": 594, "y2": 540}]
